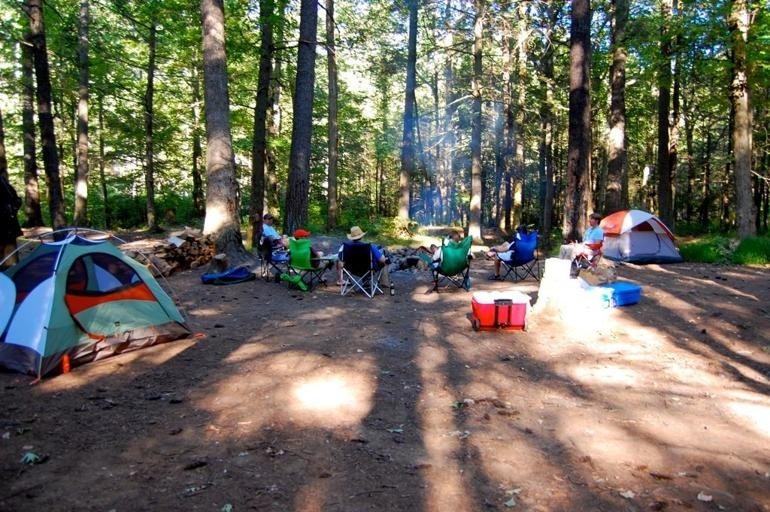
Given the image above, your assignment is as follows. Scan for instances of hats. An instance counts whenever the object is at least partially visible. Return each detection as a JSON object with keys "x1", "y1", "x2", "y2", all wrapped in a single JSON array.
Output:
[
  {"x1": 347, "y1": 226, "x2": 368, "y2": 241},
  {"x1": 294, "y1": 229, "x2": 311, "y2": 239},
  {"x1": 263, "y1": 214, "x2": 274, "y2": 220}
]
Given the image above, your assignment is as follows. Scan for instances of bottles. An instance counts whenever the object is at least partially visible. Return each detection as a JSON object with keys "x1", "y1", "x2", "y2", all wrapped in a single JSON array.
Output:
[
  {"x1": 63, "y1": 353, "x2": 70, "y2": 373},
  {"x1": 390, "y1": 281, "x2": 395, "y2": 296}
]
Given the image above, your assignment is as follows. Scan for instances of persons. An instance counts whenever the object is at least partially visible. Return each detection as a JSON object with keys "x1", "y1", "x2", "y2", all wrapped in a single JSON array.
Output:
[
  {"x1": 293, "y1": 229, "x2": 332, "y2": 284},
  {"x1": 336, "y1": 226, "x2": 390, "y2": 291},
  {"x1": 487, "y1": 224, "x2": 529, "y2": 281},
  {"x1": 560, "y1": 212, "x2": 604, "y2": 265},
  {"x1": 261, "y1": 214, "x2": 289, "y2": 248},
  {"x1": 429, "y1": 230, "x2": 477, "y2": 285}
]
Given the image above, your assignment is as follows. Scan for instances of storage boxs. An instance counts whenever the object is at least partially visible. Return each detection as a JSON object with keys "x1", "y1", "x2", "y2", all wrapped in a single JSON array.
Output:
[
  {"x1": 471, "y1": 292, "x2": 532, "y2": 332},
  {"x1": 600, "y1": 281, "x2": 641, "y2": 308}
]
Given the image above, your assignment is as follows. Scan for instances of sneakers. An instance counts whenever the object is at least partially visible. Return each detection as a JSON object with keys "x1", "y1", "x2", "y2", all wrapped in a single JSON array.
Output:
[
  {"x1": 336, "y1": 280, "x2": 351, "y2": 286},
  {"x1": 480, "y1": 250, "x2": 489, "y2": 260},
  {"x1": 488, "y1": 274, "x2": 501, "y2": 280},
  {"x1": 381, "y1": 283, "x2": 389, "y2": 288}
]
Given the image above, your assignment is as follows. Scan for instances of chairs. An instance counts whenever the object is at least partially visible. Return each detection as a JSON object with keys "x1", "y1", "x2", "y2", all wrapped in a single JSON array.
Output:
[
  {"x1": 259, "y1": 233, "x2": 327, "y2": 293},
  {"x1": 496, "y1": 230, "x2": 540, "y2": 283},
  {"x1": 341, "y1": 242, "x2": 384, "y2": 300},
  {"x1": 431, "y1": 235, "x2": 473, "y2": 294}
]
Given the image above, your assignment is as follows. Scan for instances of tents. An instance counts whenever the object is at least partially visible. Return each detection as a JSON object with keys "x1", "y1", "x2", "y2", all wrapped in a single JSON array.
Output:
[
  {"x1": 598, "y1": 208, "x2": 685, "y2": 265},
  {"x1": 1, "y1": 227, "x2": 194, "y2": 384}
]
